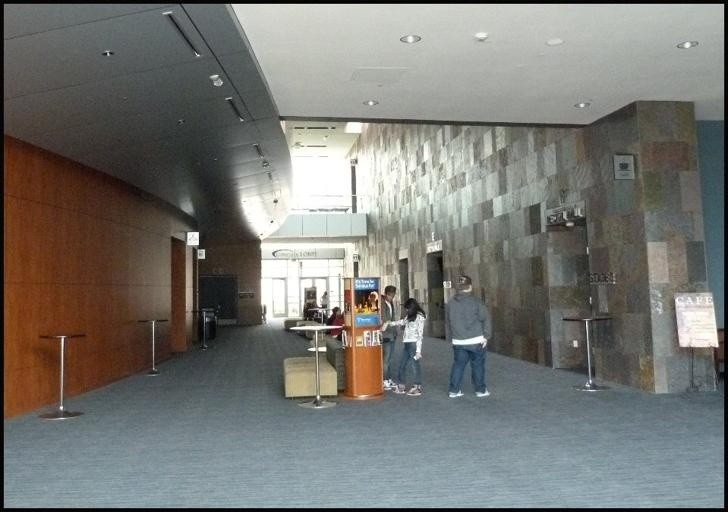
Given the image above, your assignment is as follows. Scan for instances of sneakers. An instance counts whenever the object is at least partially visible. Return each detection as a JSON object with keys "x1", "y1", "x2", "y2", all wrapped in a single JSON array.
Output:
[
  {"x1": 475, "y1": 388, "x2": 490, "y2": 397},
  {"x1": 448, "y1": 390, "x2": 464, "y2": 398},
  {"x1": 383, "y1": 379, "x2": 424, "y2": 396}
]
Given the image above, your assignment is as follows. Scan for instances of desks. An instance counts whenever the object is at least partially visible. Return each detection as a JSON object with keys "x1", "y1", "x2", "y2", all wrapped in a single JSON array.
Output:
[
  {"x1": 561, "y1": 313, "x2": 615, "y2": 392},
  {"x1": 38, "y1": 333, "x2": 84, "y2": 421},
  {"x1": 191, "y1": 309, "x2": 215, "y2": 348},
  {"x1": 137, "y1": 319, "x2": 169, "y2": 375},
  {"x1": 290, "y1": 326, "x2": 344, "y2": 409}
]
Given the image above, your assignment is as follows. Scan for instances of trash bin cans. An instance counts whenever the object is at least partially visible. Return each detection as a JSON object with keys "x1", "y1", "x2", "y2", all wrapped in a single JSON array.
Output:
[{"x1": 199, "y1": 308, "x2": 217, "y2": 340}]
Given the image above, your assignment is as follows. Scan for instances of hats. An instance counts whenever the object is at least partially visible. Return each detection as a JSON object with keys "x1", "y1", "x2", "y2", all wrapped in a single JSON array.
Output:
[{"x1": 455, "y1": 276, "x2": 472, "y2": 285}]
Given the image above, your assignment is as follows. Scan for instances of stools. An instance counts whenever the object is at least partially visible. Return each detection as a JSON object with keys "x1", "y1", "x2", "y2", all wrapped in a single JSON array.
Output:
[{"x1": 283, "y1": 357, "x2": 338, "y2": 398}]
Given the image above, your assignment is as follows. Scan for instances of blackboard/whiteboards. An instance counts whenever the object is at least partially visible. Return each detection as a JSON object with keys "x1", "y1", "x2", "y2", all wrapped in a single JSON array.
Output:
[{"x1": 198, "y1": 275, "x2": 239, "y2": 319}]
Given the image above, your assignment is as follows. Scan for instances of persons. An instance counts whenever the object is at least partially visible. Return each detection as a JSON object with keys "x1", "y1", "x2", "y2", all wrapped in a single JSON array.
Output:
[
  {"x1": 446, "y1": 276, "x2": 495, "y2": 399},
  {"x1": 389, "y1": 298, "x2": 429, "y2": 396},
  {"x1": 330, "y1": 311, "x2": 343, "y2": 342},
  {"x1": 374, "y1": 285, "x2": 399, "y2": 391},
  {"x1": 319, "y1": 291, "x2": 328, "y2": 308},
  {"x1": 325, "y1": 307, "x2": 340, "y2": 334}
]
